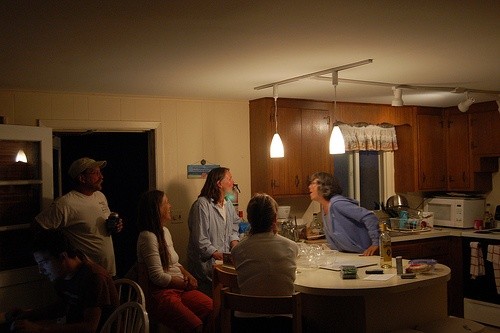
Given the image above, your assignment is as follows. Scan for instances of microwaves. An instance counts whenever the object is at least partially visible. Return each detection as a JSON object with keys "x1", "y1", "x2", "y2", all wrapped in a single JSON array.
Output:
[{"x1": 423, "y1": 198, "x2": 485, "y2": 229}]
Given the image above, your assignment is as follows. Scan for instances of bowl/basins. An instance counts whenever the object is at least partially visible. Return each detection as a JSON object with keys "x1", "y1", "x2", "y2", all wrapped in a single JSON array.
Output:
[{"x1": 320, "y1": 250, "x2": 338, "y2": 267}]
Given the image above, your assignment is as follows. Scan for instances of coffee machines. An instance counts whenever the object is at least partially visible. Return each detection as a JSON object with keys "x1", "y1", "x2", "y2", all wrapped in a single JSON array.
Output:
[{"x1": 276, "y1": 206, "x2": 291, "y2": 232}]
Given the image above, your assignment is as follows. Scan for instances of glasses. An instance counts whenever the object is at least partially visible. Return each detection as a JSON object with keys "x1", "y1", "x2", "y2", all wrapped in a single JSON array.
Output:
[
  {"x1": 83, "y1": 167, "x2": 102, "y2": 178},
  {"x1": 309, "y1": 180, "x2": 319, "y2": 187}
]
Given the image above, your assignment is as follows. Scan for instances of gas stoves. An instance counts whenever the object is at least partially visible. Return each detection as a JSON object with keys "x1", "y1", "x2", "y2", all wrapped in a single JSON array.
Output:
[{"x1": 461, "y1": 205, "x2": 500, "y2": 239}]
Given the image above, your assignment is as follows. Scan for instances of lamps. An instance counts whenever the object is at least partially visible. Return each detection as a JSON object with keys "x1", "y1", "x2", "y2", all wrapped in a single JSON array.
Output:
[
  {"x1": 457, "y1": 92, "x2": 477, "y2": 112},
  {"x1": 391, "y1": 86, "x2": 403, "y2": 106},
  {"x1": 329, "y1": 71, "x2": 345, "y2": 154},
  {"x1": 270, "y1": 84, "x2": 285, "y2": 158}
]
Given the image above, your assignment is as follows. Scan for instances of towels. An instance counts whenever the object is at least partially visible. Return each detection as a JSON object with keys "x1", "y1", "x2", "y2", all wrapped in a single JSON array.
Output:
[
  {"x1": 469, "y1": 241, "x2": 485, "y2": 279},
  {"x1": 486, "y1": 244, "x2": 500, "y2": 294}
]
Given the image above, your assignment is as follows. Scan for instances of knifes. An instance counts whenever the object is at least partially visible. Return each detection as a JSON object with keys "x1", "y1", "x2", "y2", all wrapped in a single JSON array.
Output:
[{"x1": 375, "y1": 202, "x2": 385, "y2": 212}]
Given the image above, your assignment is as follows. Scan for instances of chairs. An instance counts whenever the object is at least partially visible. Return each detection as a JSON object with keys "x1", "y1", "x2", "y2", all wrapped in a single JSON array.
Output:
[
  {"x1": 100, "y1": 278, "x2": 150, "y2": 333},
  {"x1": 211, "y1": 265, "x2": 302, "y2": 333}
]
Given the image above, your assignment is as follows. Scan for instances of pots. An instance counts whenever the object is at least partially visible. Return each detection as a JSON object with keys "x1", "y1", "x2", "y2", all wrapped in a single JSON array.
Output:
[{"x1": 387, "y1": 195, "x2": 410, "y2": 215}]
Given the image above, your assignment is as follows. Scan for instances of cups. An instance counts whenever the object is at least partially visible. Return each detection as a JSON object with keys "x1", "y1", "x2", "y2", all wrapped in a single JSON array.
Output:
[
  {"x1": 295, "y1": 243, "x2": 322, "y2": 271},
  {"x1": 473, "y1": 220, "x2": 483, "y2": 230}
]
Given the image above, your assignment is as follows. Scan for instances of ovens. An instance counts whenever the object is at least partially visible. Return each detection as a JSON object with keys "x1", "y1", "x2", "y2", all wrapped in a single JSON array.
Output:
[{"x1": 461, "y1": 237, "x2": 500, "y2": 326}]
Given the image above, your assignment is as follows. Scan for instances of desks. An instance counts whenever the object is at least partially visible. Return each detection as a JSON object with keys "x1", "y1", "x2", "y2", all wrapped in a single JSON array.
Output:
[{"x1": 295, "y1": 245, "x2": 452, "y2": 333}]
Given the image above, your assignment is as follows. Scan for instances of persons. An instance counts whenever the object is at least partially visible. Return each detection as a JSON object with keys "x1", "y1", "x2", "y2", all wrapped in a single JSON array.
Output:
[
  {"x1": 308, "y1": 171, "x2": 382, "y2": 256},
  {"x1": 29, "y1": 157, "x2": 124, "y2": 282},
  {"x1": 187, "y1": 167, "x2": 241, "y2": 333},
  {"x1": 135, "y1": 190, "x2": 220, "y2": 333},
  {"x1": 231, "y1": 193, "x2": 299, "y2": 333},
  {"x1": 4, "y1": 229, "x2": 120, "y2": 333}
]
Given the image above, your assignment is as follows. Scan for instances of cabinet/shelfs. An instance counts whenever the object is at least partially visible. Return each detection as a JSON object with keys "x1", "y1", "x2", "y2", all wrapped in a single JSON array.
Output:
[
  {"x1": 389, "y1": 236, "x2": 500, "y2": 329},
  {"x1": 249, "y1": 97, "x2": 500, "y2": 200}
]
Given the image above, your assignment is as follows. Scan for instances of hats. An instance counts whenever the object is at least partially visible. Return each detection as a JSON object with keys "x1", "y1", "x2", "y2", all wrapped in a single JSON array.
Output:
[{"x1": 70, "y1": 158, "x2": 107, "y2": 178}]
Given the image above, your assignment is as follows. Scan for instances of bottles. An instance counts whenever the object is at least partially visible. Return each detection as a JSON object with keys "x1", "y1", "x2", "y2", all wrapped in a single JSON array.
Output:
[
  {"x1": 379, "y1": 221, "x2": 392, "y2": 269},
  {"x1": 309, "y1": 213, "x2": 321, "y2": 234}
]
